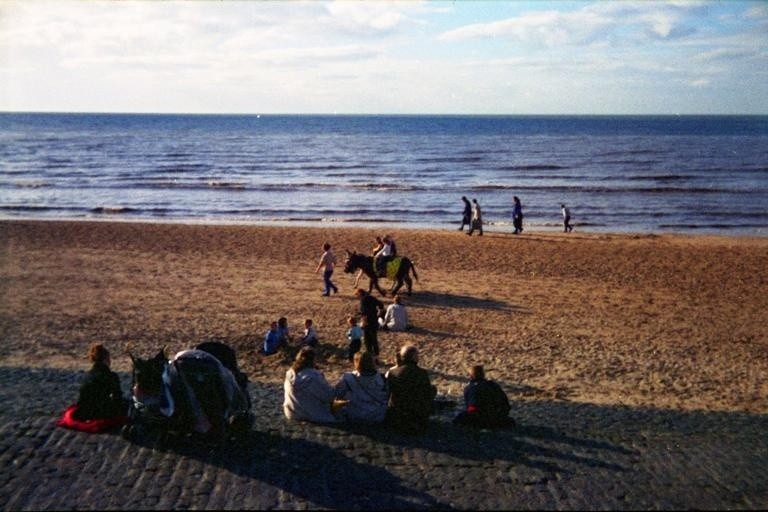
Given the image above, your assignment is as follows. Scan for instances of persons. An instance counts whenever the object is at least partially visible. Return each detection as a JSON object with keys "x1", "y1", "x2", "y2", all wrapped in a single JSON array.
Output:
[
  {"x1": 336, "y1": 352, "x2": 390, "y2": 422},
  {"x1": 511, "y1": 196, "x2": 523, "y2": 234},
  {"x1": 458, "y1": 196, "x2": 472, "y2": 232},
  {"x1": 384, "y1": 344, "x2": 438, "y2": 419},
  {"x1": 452, "y1": 365, "x2": 516, "y2": 430},
  {"x1": 282, "y1": 346, "x2": 338, "y2": 423},
  {"x1": 264, "y1": 235, "x2": 408, "y2": 362},
  {"x1": 72, "y1": 344, "x2": 130, "y2": 422},
  {"x1": 465, "y1": 198, "x2": 483, "y2": 236},
  {"x1": 560, "y1": 204, "x2": 573, "y2": 233}
]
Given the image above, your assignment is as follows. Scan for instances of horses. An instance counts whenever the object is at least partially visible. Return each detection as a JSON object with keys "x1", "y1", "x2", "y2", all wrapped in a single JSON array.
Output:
[{"x1": 343, "y1": 249, "x2": 419, "y2": 297}]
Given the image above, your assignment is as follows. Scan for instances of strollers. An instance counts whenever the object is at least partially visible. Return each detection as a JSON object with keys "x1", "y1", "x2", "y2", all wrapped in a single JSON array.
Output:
[
  {"x1": 117, "y1": 343, "x2": 178, "y2": 452},
  {"x1": 153, "y1": 335, "x2": 257, "y2": 461}
]
